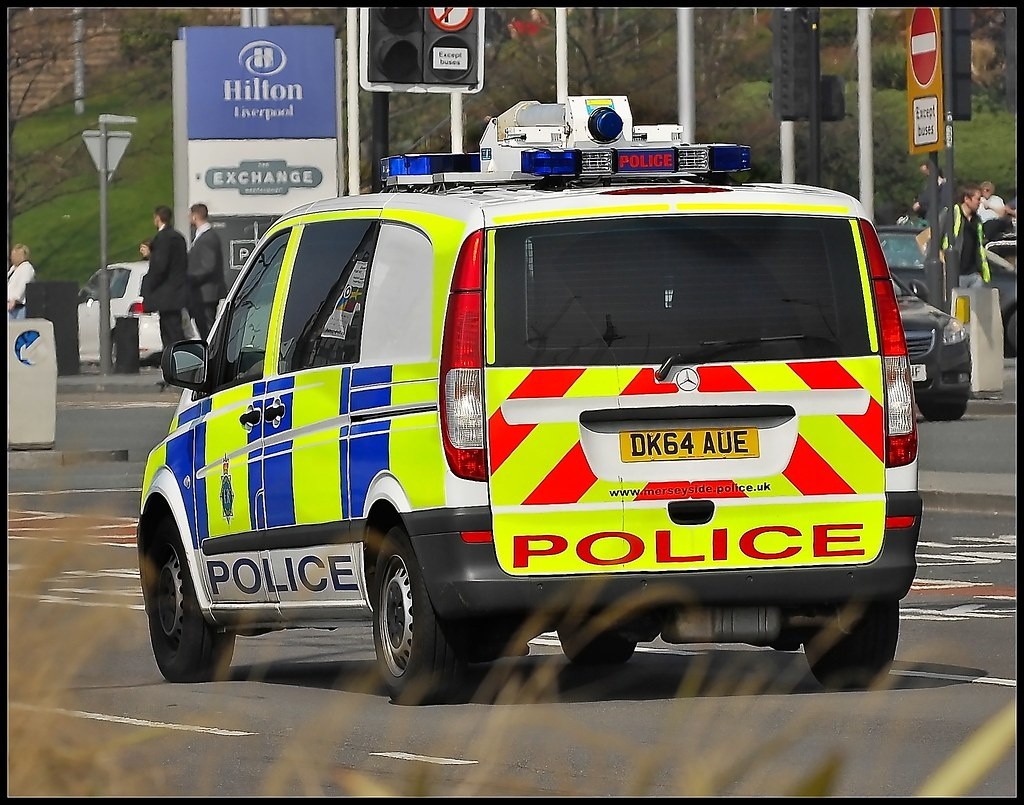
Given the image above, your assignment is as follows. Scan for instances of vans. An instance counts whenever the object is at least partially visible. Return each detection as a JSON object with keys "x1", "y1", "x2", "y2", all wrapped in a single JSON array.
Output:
[{"x1": 136, "y1": 92, "x2": 924, "y2": 705}]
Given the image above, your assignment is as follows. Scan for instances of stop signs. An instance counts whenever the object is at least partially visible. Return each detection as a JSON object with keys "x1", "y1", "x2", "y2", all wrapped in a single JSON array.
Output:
[{"x1": 908, "y1": 7, "x2": 939, "y2": 88}]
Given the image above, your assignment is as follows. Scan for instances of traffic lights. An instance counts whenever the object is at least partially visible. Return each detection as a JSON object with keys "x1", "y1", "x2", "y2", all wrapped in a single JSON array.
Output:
[{"x1": 366, "y1": 8, "x2": 422, "y2": 85}]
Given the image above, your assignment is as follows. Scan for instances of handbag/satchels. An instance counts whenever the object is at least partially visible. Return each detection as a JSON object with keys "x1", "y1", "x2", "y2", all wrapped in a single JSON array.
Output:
[{"x1": 916, "y1": 206, "x2": 948, "y2": 262}]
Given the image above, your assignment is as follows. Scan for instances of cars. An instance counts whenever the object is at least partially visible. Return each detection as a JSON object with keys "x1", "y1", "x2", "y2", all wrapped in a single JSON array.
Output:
[
  {"x1": 76, "y1": 260, "x2": 201, "y2": 369},
  {"x1": 868, "y1": 227, "x2": 1016, "y2": 422}
]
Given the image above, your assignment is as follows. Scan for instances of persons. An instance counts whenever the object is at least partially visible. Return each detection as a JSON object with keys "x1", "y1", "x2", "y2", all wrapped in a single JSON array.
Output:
[
  {"x1": 913, "y1": 160, "x2": 1017, "y2": 288},
  {"x1": 7, "y1": 244, "x2": 37, "y2": 320},
  {"x1": 187, "y1": 204, "x2": 228, "y2": 340},
  {"x1": 140, "y1": 206, "x2": 189, "y2": 387}
]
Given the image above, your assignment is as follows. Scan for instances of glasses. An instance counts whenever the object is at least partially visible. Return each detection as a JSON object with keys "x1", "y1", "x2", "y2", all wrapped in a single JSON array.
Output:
[{"x1": 986, "y1": 188, "x2": 990, "y2": 192}]
